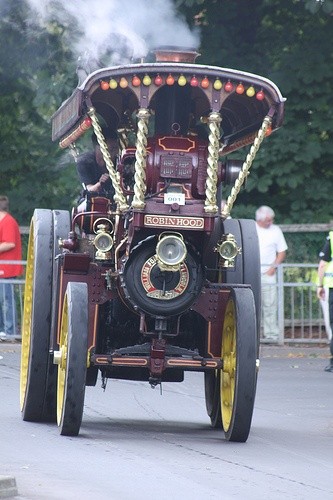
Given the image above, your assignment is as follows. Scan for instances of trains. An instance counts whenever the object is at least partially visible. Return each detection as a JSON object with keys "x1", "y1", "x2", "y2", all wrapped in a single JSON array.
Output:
[{"x1": 18, "y1": 33, "x2": 287, "y2": 440}]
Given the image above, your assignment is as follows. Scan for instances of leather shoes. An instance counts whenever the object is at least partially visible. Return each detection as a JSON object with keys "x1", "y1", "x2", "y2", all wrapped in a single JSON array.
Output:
[{"x1": 324, "y1": 364, "x2": 333, "y2": 372}]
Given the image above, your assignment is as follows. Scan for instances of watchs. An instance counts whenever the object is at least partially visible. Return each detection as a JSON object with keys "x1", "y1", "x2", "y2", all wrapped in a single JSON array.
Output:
[{"x1": 270, "y1": 264, "x2": 278, "y2": 273}]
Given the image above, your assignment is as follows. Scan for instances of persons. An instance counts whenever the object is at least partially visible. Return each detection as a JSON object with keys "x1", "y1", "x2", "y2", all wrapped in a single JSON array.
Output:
[
  {"x1": 254, "y1": 206, "x2": 288, "y2": 344},
  {"x1": 316, "y1": 211, "x2": 333, "y2": 372},
  {"x1": 0, "y1": 195, "x2": 25, "y2": 342}
]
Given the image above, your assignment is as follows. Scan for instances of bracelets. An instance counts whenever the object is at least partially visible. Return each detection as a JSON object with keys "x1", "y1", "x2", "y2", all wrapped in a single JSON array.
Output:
[{"x1": 317, "y1": 286, "x2": 323, "y2": 288}]
